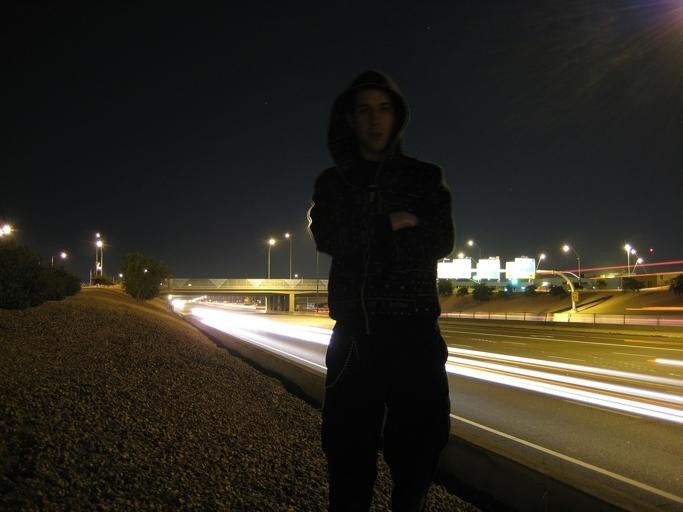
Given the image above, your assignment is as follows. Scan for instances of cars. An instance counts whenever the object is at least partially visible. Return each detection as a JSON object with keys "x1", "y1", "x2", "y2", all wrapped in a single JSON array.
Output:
[{"x1": 451, "y1": 283, "x2": 554, "y2": 294}]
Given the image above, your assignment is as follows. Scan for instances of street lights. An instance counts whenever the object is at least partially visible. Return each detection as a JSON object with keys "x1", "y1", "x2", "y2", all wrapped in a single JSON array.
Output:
[
  {"x1": 467, "y1": 240, "x2": 481, "y2": 259},
  {"x1": 536, "y1": 253, "x2": 545, "y2": 269},
  {"x1": 623, "y1": 243, "x2": 647, "y2": 274},
  {"x1": 266, "y1": 238, "x2": 275, "y2": 278},
  {"x1": 94, "y1": 232, "x2": 100, "y2": 276},
  {"x1": 50, "y1": 252, "x2": 67, "y2": 268},
  {"x1": 284, "y1": 233, "x2": 293, "y2": 279},
  {"x1": 96, "y1": 241, "x2": 104, "y2": 277},
  {"x1": 561, "y1": 243, "x2": 581, "y2": 287}
]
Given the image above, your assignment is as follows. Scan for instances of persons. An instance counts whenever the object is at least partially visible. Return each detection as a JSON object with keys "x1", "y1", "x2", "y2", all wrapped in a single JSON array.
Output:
[{"x1": 307, "y1": 69, "x2": 455, "y2": 512}]
